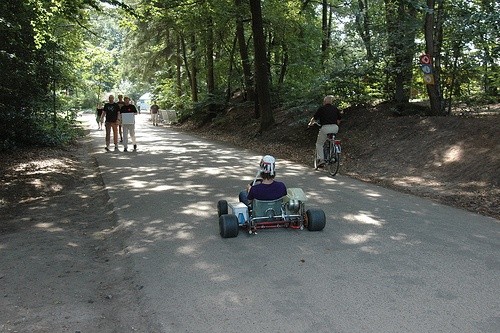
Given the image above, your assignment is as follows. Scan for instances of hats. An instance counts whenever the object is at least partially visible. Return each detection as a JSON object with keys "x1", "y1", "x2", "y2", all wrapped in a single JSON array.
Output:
[{"x1": 260, "y1": 155, "x2": 276, "y2": 176}]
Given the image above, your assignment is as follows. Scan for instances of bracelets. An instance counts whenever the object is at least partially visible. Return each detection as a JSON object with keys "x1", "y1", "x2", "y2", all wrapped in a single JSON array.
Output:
[{"x1": 118, "y1": 119, "x2": 120, "y2": 121}]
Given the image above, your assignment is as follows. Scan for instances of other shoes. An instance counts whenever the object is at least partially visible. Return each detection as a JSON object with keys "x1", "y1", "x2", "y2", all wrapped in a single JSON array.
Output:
[
  {"x1": 124, "y1": 148, "x2": 127, "y2": 151},
  {"x1": 133, "y1": 149, "x2": 136, "y2": 152},
  {"x1": 115, "y1": 147, "x2": 119, "y2": 151},
  {"x1": 105, "y1": 147, "x2": 111, "y2": 152},
  {"x1": 317, "y1": 160, "x2": 325, "y2": 166}
]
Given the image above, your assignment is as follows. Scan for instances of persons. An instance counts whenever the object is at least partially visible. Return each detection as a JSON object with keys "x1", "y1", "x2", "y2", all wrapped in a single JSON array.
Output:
[
  {"x1": 136, "y1": 100, "x2": 141, "y2": 114},
  {"x1": 120, "y1": 97, "x2": 137, "y2": 152},
  {"x1": 239, "y1": 155, "x2": 287, "y2": 220},
  {"x1": 95, "y1": 103, "x2": 106, "y2": 131},
  {"x1": 150, "y1": 101, "x2": 160, "y2": 126},
  {"x1": 309, "y1": 95, "x2": 341, "y2": 167},
  {"x1": 116, "y1": 94, "x2": 125, "y2": 142},
  {"x1": 101, "y1": 95, "x2": 120, "y2": 151}
]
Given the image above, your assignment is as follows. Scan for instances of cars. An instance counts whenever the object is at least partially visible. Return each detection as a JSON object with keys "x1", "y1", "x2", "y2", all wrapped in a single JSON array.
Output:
[{"x1": 135, "y1": 92, "x2": 162, "y2": 114}]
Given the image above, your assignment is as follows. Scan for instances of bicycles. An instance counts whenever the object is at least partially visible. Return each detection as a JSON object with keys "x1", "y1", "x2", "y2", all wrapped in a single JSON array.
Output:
[{"x1": 308, "y1": 121, "x2": 342, "y2": 176}]
